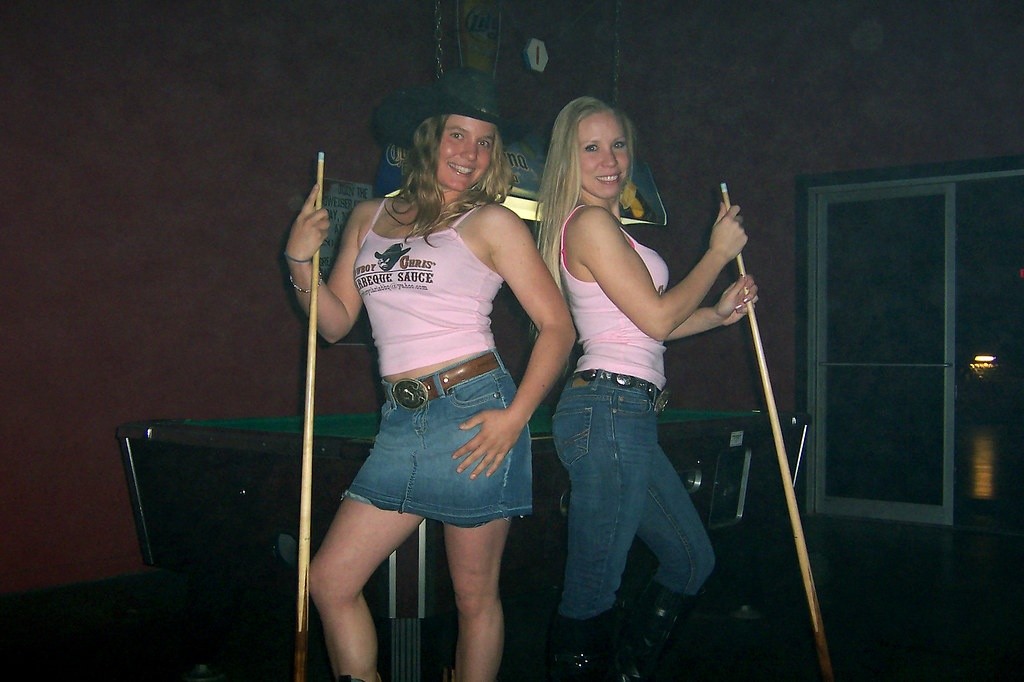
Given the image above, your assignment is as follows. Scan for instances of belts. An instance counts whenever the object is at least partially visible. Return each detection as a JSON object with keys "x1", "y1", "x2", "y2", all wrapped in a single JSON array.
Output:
[
  {"x1": 382, "y1": 353, "x2": 500, "y2": 410},
  {"x1": 580, "y1": 370, "x2": 670, "y2": 414}
]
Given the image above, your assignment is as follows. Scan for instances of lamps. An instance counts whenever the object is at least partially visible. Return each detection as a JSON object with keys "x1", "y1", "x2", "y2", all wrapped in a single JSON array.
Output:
[{"x1": 378, "y1": 0, "x2": 667, "y2": 228}]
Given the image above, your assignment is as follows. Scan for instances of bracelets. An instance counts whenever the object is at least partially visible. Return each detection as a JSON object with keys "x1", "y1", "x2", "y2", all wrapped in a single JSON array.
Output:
[
  {"x1": 287, "y1": 255, "x2": 312, "y2": 263},
  {"x1": 289, "y1": 271, "x2": 323, "y2": 293}
]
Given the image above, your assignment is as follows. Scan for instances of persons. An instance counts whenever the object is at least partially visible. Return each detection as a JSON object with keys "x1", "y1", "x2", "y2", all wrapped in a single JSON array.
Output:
[
  {"x1": 531, "y1": 95, "x2": 759, "y2": 682},
  {"x1": 285, "y1": 71, "x2": 577, "y2": 682}
]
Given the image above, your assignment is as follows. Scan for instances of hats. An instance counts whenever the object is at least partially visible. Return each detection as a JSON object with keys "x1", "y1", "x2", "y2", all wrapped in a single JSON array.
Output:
[{"x1": 378, "y1": 67, "x2": 530, "y2": 148}]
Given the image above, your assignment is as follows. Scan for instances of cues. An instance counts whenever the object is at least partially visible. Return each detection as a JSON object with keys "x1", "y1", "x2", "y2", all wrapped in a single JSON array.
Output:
[
  {"x1": 293, "y1": 147, "x2": 327, "y2": 681},
  {"x1": 718, "y1": 180, "x2": 839, "y2": 680}
]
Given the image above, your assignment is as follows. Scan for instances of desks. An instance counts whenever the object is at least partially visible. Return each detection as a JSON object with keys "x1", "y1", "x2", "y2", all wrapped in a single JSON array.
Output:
[{"x1": 113, "y1": 405, "x2": 814, "y2": 682}]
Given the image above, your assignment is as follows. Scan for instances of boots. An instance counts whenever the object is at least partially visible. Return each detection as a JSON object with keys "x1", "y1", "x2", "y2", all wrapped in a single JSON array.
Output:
[
  {"x1": 546, "y1": 635, "x2": 617, "y2": 682},
  {"x1": 607, "y1": 579, "x2": 707, "y2": 682}
]
